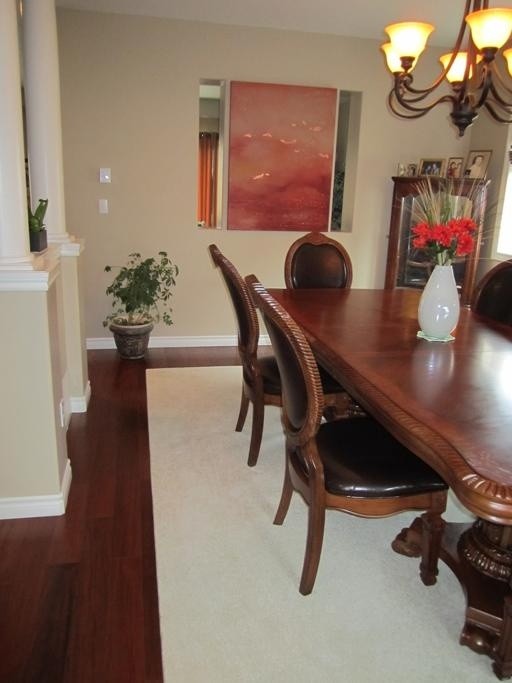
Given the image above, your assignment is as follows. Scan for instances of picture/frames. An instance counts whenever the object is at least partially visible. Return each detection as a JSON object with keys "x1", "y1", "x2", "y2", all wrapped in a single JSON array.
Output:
[{"x1": 418, "y1": 150, "x2": 493, "y2": 180}]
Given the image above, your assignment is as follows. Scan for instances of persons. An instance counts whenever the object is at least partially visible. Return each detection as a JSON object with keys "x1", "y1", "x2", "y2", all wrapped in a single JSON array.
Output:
[
  {"x1": 423, "y1": 162, "x2": 440, "y2": 175},
  {"x1": 468, "y1": 156, "x2": 484, "y2": 177},
  {"x1": 448, "y1": 160, "x2": 461, "y2": 178}
]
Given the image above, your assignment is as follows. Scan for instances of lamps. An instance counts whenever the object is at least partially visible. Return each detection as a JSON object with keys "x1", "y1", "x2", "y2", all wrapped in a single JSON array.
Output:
[{"x1": 380, "y1": 0, "x2": 512, "y2": 137}]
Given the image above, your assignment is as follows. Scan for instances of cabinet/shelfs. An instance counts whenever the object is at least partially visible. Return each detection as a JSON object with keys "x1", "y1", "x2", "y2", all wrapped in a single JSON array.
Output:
[{"x1": 385, "y1": 177, "x2": 491, "y2": 311}]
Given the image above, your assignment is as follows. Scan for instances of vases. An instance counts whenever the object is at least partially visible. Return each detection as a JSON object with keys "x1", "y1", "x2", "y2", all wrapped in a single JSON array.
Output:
[{"x1": 417, "y1": 265, "x2": 460, "y2": 340}]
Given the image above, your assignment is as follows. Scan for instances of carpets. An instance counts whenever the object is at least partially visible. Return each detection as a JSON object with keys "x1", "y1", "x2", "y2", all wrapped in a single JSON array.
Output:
[{"x1": 146, "y1": 365, "x2": 512, "y2": 683}]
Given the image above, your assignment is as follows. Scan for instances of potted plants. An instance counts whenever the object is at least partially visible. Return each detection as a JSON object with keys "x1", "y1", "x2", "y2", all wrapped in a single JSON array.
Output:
[
  {"x1": 103, "y1": 251, "x2": 179, "y2": 359},
  {"x1": 29, "y1": 198, "x2": 48, "y2": 252}
]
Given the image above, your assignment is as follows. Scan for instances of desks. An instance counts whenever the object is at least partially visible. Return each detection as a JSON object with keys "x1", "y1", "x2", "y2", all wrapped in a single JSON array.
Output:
[{"x1": 266, "y1": 289, "x2": 512, "y2": 680}]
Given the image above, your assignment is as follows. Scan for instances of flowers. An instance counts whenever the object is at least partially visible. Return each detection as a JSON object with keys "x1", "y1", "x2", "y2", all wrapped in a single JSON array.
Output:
[{"x1": 389, "y1": 176, "x2": 512, "y2": 267}]
